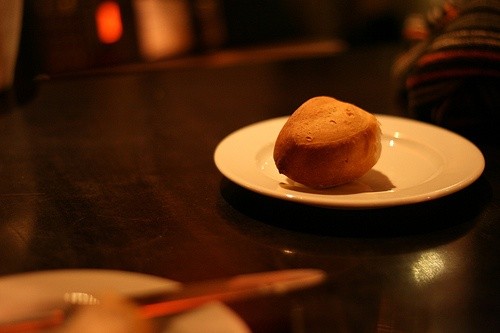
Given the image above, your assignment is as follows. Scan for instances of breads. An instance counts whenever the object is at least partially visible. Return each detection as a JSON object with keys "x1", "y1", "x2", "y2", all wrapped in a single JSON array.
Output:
[{"x1": 272, "y1": 97, "x2": 383, "y2": 189}]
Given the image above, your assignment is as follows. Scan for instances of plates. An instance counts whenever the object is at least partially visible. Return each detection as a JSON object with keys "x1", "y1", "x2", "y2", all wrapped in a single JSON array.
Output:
[
  {"x1": 1, "y1": 269, "x2": 251, "y2": 333},
  {"x1": 213, "y1": 114, "x2": 486, "y2": 207}
]
